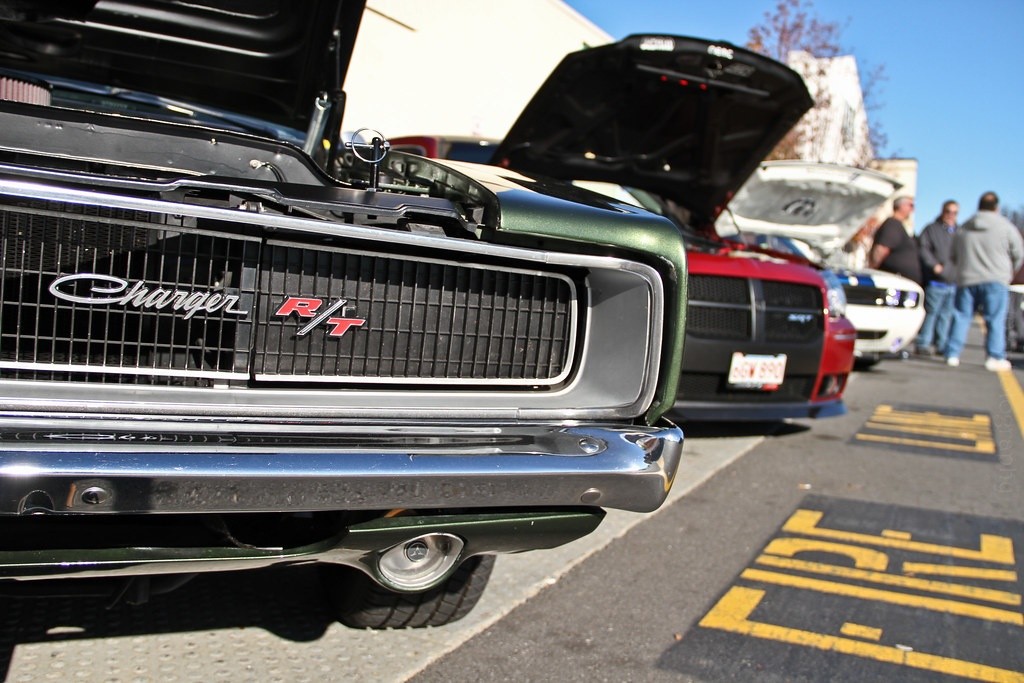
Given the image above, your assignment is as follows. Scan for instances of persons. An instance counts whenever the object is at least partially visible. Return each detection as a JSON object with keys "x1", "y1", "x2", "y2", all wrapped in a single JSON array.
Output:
[
  {"x1": 868, "y1": 196, "x2": 922, "y2": 359},
  {"x1": 945, "y1": 192, "x2": 1024, "y2": 373},
  {"x1": 915, "y1": 201, "x2": 960, "y2": 356}
]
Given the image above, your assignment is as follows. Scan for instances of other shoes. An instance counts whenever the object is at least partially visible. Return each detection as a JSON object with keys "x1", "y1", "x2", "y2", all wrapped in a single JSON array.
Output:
[
  {"x1": 945, "y1": 356, "x2": 960, "y2": 366},
  {"x1": 984, "y1": 356, "x2": 1013, "y2": 372},
  {"x1": 916, "y1": 346, "x2": 931, "y2": 356},
  {"x1": 935, "y1": 350, "x2": 948, "y2": 357}
]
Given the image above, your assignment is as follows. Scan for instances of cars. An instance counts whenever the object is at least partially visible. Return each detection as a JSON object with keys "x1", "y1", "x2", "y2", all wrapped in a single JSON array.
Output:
[
  {"x1": 364, "y1": 33, "x2": 856, "y2": 429},
  {"x1": 0, "y1": 0, "x2": 689, "y2": 628},
  {"x1": 716, "y1": 159, "x2": 927, "y2": 370}
]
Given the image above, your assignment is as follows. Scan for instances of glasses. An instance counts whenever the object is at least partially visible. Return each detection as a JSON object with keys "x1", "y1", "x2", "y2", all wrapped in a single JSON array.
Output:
[
  {"x1": 905, "y1": 203, "x2": 914, "y2": 208},
  {"x1": 945, "y1": 210, "x2": 958, "y2": 216}
]
